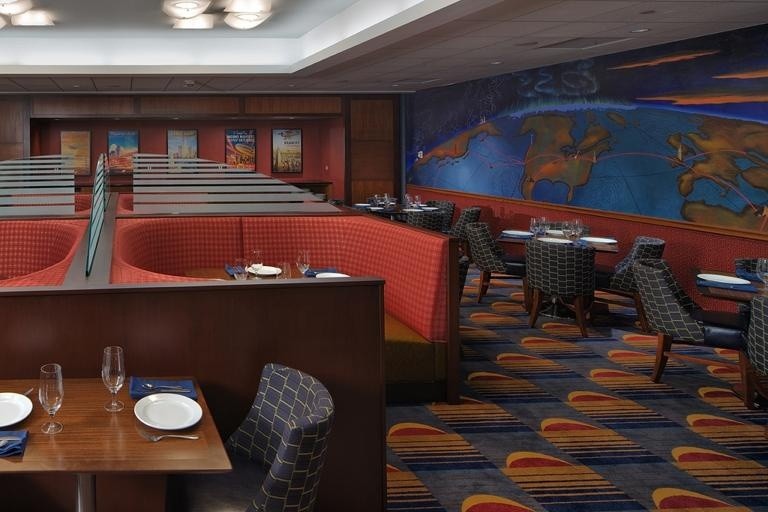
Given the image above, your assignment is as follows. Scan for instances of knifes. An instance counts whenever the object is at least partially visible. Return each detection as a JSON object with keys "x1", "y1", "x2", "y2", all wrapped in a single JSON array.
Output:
[{"x1": 0, "y1": 436, "x2": 21, "y2": 441}]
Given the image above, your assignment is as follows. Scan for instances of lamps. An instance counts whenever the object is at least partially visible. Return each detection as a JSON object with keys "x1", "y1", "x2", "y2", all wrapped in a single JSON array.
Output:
[
  {"x1": 0, "y1": 0, "x2": 55, "y2": 30},
  {"x1": 161, "y1": 0, "x2": 273, "y2": 30}
]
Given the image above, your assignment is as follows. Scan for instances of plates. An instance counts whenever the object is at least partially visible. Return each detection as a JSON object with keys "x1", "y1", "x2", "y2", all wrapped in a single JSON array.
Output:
[
  {"x1": 133, "y1": 393, "x2": 204, "y2": 431},
  {"x1": 402, "y1": 202, "x2": 440, "y2": 212},
  {"x1": 316, "y1": 272, "x2": 351, "y2": 278},
  {"x1": 537, "y1": 237, "x2": 573, "y2": 244},
  {"x1": 696, "y1": 272, "x2": 751, "y2": 286},
  {"x1": 501, "y1": 230, "x2": 533, "y2": 238},
  {"x1": 247, "y1": 266, "x2": 282, "y2": 276},
  {"x1": 354, "y1": 200, "x2": 396, "y2": 213},
  {"x1": 545, "y1": 229, "x2": 576, "y2": 236},
  {"x1": 580, "y1": 237, "x2": 618, "y2": 244},
  {"x1": 0, "y1": 391, "x2": 33, "y2": 428}
]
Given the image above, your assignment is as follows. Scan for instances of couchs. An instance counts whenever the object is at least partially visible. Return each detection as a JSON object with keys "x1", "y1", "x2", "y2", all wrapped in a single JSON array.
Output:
[{"x1": 0, "y1": 193, "x2": 460, "y2": 405}]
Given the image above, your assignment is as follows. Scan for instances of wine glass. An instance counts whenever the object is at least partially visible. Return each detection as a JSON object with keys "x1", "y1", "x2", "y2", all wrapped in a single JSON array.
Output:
[
  {"x1": 39, "y1": 364, "x2": 65, "y2": 434},
  {"x1": 540, "y1": 216, "x2": 550, "y2": 237},
  {"x1": 756, "y1": 258, "x2": 768, "y2": 292},
  {"x1": 249, "y1": 249, "x2": 264, "y2": 280},
  {"x1": 102, "y1": 346, "x2": 126, "y2": 412},
  {"x1": 373, "y1": 192, "x2": 391, "y2": 210},
  {"x1": 404, "y1": 193, "x2": 422, "y2": 210},
  {"x1": 562, "y1": 218, "x2": 585, "y2": 241},
  {"x1": 296, "y1": 250, "x2": 311, "y2": 278},
  {"x1": 530, "y1": 218, "x2": 541, "y2": 239}
]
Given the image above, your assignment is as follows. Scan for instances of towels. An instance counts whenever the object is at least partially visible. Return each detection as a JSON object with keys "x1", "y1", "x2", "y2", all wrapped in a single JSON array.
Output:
[{"x1": 0, "y1": 430, "x2": 29, "y2": 457}]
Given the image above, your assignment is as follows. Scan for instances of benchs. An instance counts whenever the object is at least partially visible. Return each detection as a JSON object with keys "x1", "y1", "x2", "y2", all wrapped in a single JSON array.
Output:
[{"x1": 75, "y1": 178, "x2": 332, "y2": 191}]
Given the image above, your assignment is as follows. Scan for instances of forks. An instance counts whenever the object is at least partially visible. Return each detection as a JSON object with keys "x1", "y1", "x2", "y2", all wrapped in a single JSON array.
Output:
[
  {"x1": 137, "y1": 428, "x2": 199, "y2": 442},
  {"x1": 135, "y1": 387, "x2": 192, "y2": 395}
]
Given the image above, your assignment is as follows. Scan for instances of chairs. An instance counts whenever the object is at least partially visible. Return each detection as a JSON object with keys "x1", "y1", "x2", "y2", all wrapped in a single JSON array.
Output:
[
  {"x1": 742, "y1": 295, "x2": 767, "y2": 408},
  {"x1": 735, "y1": 258, "x2": 758, "y2": 312},
  {"x1": 461, "y1": 207, "x2": 667, "y2": 337},
  {"x1": 633, "y1": 259, "x2": 746, "y2": 382},
  {"x1": 182, "y1": 362, "x2": 337, "y2": 511}
]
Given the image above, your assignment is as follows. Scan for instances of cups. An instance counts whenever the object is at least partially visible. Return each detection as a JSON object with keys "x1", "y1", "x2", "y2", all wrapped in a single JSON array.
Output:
[
  {"x1": 276, "y1": 262, "x2": 292, "y2": 279},
  {"x1": 233, "y1": 258, "x2": 249, "y2": 281}
]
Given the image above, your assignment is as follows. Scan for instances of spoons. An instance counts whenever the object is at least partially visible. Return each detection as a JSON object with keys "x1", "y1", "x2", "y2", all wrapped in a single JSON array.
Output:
[{"x1": 142, "y1": 384, "x2": 184, "y2": 389}]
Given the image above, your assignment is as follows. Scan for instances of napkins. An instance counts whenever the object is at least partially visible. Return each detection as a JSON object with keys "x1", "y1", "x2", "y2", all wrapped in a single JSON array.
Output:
[
  {"x1": 696, "y1": 278, "x2": 758, "y2": 292},
  {"x1": 735, "y1": 269, "x2": 761, "y2": 283}
]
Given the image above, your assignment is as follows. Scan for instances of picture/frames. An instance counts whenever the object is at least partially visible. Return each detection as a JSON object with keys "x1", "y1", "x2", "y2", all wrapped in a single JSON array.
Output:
[
  {"x1": 107, "y1": 128, "x2": 141, "y2": 177},
  {"x1": 224, "y1": 128, "x2": 257, "y2": 170},
  {"x1": 166, "y1": 129, "x2": 199, "y2": 174},
  {"x1": 271, "y1": 128, "x2": 303, "y2": 173},
  {"x1": 60, "y1": 129, "x2": 91, "y2": 176}
]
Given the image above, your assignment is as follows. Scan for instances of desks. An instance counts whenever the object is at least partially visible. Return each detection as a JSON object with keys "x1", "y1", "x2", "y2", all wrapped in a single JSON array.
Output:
[{"x1": 0, "y1": 376, "x2": 233, "y2": 512}]
